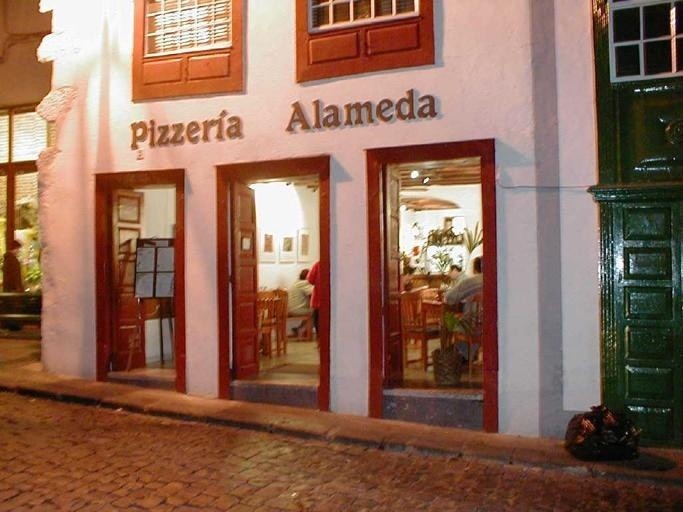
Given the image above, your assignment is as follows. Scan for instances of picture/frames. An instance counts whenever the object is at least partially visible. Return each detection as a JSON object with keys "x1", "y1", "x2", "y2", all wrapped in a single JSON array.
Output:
[{"x1": 259, "y1": 225, "x2": 314, "y2": 264}]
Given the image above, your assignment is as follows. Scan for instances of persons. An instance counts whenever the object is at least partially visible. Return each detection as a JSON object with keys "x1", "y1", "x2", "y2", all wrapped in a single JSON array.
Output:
[
  {"x1": 443, "y1": 255, "x2": 482, "y2": 365},
  {"x1": 442, "y1": 264, "x2": 462, "y2": 288},
  {"x1": 305, "y1": 260, "x2": 321, "y2": 344},
  {"x1": 0, "y1": 240, "x2": 24, "y2": 332},
  {"x1": 286, "y1": 269, "x2": 315, "y2": 341},
  {"x1": 397, "y1": 267, "x2": 414, "y2": 294}
]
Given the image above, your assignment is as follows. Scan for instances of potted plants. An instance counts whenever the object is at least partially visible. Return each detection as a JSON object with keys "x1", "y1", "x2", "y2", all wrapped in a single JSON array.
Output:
[{"x1": 431, "y1": 220, "x2": 485, "y2": 388}]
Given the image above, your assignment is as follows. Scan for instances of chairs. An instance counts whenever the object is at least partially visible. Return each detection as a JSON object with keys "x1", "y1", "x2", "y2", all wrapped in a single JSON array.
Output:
[
  {"x1": 400, "y1": 282, "x2": 486, "y2": 382},
  {"x1": 256, "y1": 285, "x2": 290, "y2": 362}
]
